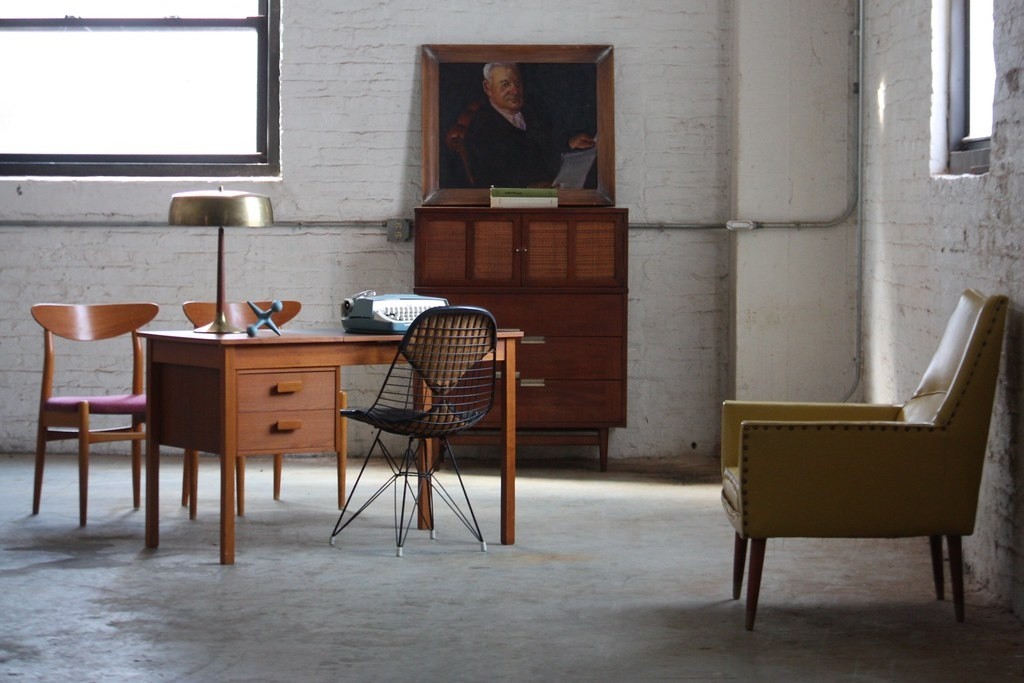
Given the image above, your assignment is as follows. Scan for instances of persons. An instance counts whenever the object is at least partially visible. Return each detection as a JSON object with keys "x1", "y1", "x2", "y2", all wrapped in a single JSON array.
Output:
[{"x1": 465, "y1": 62, "x2": 598, "y2": 188}]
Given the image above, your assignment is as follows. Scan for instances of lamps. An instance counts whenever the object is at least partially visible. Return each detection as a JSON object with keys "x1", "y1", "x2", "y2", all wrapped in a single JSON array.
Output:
[{"x1": 168, "y1": 185, "x2": 275, "y2": 333}]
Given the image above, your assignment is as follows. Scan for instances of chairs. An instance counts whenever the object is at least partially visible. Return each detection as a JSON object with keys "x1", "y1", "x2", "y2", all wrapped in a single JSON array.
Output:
[
  {"x1": 330, "y1": 304, "x2": 499, "y2": 557},
  {"x1": 180, "y1": 300, "x2": 348, "y2": 518},
  {"x1": 30, "y1": 301, "x2": 197, "y2": 527},
  {"x1": 721, "y1": 289, "x2": 1009, "y2": 632}
]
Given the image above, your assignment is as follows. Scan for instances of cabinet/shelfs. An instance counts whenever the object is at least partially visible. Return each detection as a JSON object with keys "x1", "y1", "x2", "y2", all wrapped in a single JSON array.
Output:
[{"x1": 411, "y1": 205, "x2": 630, "y2": 471}]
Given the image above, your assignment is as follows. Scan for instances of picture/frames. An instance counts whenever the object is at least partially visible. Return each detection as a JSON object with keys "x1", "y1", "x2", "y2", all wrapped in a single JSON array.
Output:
[{"x1": 422, "y1": 42, "x2": 615, "y2": 205}]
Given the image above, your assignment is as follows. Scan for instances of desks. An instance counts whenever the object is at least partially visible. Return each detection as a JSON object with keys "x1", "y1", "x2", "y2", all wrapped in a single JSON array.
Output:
[{"x1": 135, "y1": 329, "x2": 524, "y2": 565}]
